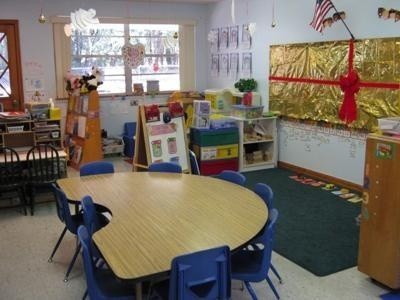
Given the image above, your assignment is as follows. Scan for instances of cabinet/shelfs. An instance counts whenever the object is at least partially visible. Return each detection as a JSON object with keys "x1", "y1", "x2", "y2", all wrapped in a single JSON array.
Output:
[
  {"x1": 64, "y1": 91, "x2": 102, "y2": 171},
  {"x1": 227, "y1": 115, "x2": 278, "y2": 172},
  {"x1": 0, "y1": 118, "x2": 62, "y2": 152}
]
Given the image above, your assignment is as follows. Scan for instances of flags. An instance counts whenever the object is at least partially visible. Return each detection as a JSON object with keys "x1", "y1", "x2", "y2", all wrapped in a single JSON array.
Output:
[{"x1": 308, "y1": 0, "x2": 334, "y2": 35}]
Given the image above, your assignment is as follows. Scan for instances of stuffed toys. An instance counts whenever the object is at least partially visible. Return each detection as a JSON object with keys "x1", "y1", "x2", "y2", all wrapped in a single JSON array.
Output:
[{"x1": 63, "y1": 66, "x2": 105, "y2": 97}]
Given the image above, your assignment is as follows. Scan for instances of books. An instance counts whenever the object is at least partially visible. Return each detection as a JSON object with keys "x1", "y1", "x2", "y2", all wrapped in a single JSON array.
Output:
[{"x1": 64, "y1": 93, "x2": 91, "y2": 164}]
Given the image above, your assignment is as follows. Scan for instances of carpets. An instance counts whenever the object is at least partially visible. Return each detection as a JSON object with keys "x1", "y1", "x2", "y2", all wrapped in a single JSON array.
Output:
[{"x1": 211, "y1": 167, "x2": 364, "y2": 277}]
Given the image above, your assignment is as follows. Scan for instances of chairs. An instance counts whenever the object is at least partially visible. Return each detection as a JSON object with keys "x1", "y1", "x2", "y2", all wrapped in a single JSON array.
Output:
[
  {"x1": 0, "y1": 144, "x2": 60, "y2": 216},
  {"x1": 48, "y1": 162, "x2": 283, "y2": 300}
]
